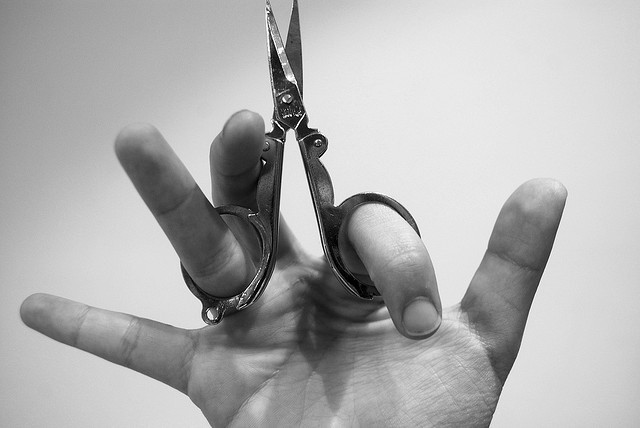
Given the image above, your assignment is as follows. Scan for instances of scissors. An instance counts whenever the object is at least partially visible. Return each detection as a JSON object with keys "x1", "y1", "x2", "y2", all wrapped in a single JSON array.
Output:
[{"x1": 179, "y1": 0, "x2": 424, "y2": 327}]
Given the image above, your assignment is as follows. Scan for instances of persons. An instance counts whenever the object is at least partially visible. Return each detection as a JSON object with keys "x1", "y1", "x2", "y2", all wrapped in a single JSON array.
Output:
[{"x1": 20, "y1": 110, "x2": 567, "y2": 428}]
